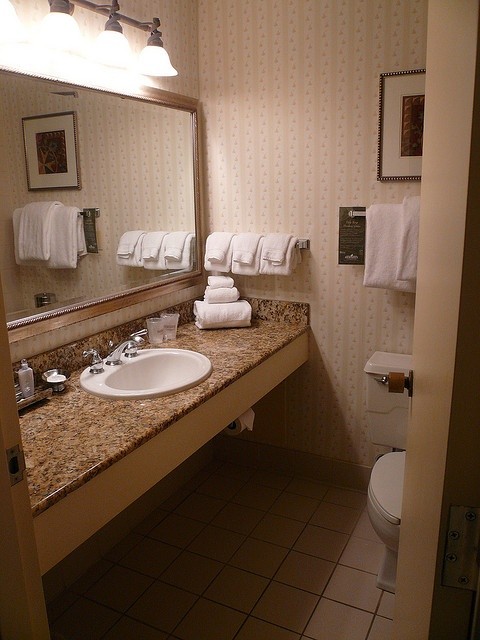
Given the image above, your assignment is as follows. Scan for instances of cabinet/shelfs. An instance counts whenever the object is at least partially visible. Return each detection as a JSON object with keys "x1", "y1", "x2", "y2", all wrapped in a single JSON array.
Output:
[
  {"x1": 77, "y1": 207, "x2": 100, "y2": 253},
  {"x1": 296, "y1": 239, "x2": 310, "y2": 250}
]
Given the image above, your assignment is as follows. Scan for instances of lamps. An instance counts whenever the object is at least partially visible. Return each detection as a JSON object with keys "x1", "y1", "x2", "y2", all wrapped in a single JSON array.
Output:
[{"x1": 0, "y1": 1, "x2": 178, "y2": 78}]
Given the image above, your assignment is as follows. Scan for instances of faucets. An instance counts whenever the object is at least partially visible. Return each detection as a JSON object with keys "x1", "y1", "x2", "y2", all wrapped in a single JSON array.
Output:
[{"x1": 106, "y1": 340, "x2": 137, "y2": 365}]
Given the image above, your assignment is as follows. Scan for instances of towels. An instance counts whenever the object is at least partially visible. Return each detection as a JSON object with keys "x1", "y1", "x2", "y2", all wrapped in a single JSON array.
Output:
[
  {"x1": 396, "y1": 195, "x2": 421, "y2": 282},
  {"x1": 207, "y1": 275, "x2": 235, "y2": 289},
  {"x1": 116, "y1": 231, "x2": 146, "y2": 268},
  {"x1": 205, "y1": 232, "x2": 235, "y2": 265},
  {"x1": 164, "y1": 230, "x2": 190, "y2": 263},
  {"x1": 203, "y1": 286, "x2": 240, "y2": 303},
  {"x1": 362, "y1": 204, "x2": 416, "y2": 295},
  {"x1": 204, "y1": 231, "x2": 237, "y2": 273},
  {"x1": 193, "y1": 301, "x2": 252, "y2": 329},
  {"x1": 18, "y1": 201, "x2": 64, "y2": 262},
  {"x1": 12, "y1": 206, "x2": 88, "y2": 269},
  {"x1": 141, "y1": 231, "x2": 169, "y2": 262},
  {"x1": 259, "y1": 234, "x2": 302, "y2": 277},
  {"x1": 116, "y1": 230, "x2": 144, "y2": 260},
  {"x1": 141, "y1": 231, "x2": 170, "y2": 271},
  {"x1": 231, "y1": 233, "x2": 265, "y2": 277},
  {"x1": 261, "y1": 234, "x2": 294, "y2": 267},
  {"x1": 164, "y1": 232, "x2": 195, "y2": 270},
  {"x1": 232, "y1": 234, "x2": 264, "y2": 266}
]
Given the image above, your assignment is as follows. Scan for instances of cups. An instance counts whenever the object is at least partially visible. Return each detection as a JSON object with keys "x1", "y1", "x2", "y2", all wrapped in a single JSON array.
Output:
[
  {"x1": 148, "y1": 318, "x2": 164, "y2": 343},
  {"x1": 161, "y1": 313, "x2": 178, "y2": 339}
]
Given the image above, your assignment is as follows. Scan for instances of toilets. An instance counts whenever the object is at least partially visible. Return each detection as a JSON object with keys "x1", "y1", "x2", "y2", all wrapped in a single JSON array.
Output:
[{"x1": 364, "y1": 351, "x2": 413, "y2": 594}]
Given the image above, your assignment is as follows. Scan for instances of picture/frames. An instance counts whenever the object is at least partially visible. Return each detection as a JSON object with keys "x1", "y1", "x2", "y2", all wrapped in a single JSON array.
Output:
[
  {"x1": 22, "y1": 111, "x2": 83, "y2": 192},
  {"x1": 376, "y1": 68, "x2": 427, "y2": 181}
]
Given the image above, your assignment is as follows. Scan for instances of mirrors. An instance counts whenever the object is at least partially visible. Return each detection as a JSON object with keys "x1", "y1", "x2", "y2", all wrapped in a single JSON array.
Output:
[{"x1": 0, "y1": 59, "x2": 209, "y2": 344}]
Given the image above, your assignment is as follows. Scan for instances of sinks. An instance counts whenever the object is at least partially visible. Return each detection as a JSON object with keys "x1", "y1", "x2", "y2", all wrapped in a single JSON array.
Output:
[{"x1": 80, "y1": 348, "x2": 212, "y2": 399}]
